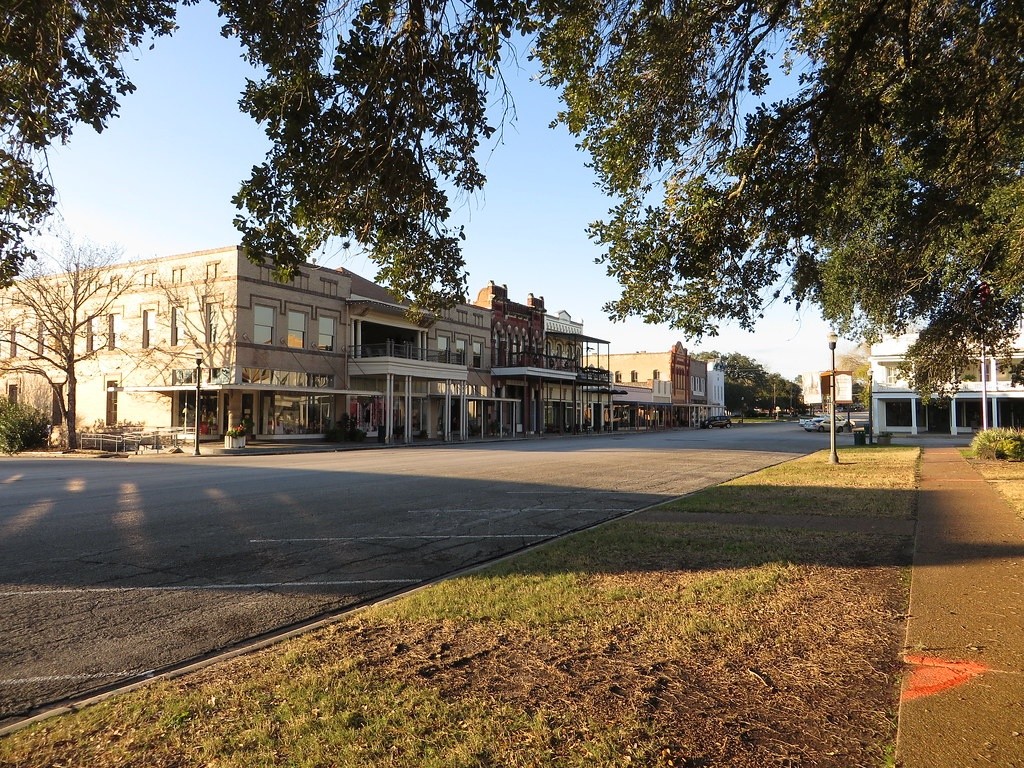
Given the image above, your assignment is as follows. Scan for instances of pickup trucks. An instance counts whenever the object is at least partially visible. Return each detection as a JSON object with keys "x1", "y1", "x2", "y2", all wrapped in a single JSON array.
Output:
[{"x1": 799, "y1": 414, "x2": 856, "y2": 429}]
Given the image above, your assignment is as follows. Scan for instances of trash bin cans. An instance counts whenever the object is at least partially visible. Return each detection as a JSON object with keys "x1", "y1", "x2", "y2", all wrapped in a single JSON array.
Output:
[
  {"x1": 864, "y1": 424, "x2": 869, "y2": 433},
  {"x1": 853, "y1": 430, "x2": 866, "y2": 446},
  {"x1": 613, "y1": 421, "x2": 619, "y2": 431}
]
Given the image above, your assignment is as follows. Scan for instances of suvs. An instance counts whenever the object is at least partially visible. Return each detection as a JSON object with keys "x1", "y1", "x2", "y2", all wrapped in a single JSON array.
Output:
[{"x1": 699, "y1": 415, "x2": 732, "y2": 429}]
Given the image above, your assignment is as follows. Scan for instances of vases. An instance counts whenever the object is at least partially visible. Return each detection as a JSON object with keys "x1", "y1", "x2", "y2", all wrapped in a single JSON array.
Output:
[{"x1": 225, "y1": 436, "x2": 246, "y2": 449}]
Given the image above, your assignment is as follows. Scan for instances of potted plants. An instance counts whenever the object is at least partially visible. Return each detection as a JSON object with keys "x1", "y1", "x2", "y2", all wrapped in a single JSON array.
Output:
[{"x1": 877, "y1": 432, "x2": 893, "y2": 445}]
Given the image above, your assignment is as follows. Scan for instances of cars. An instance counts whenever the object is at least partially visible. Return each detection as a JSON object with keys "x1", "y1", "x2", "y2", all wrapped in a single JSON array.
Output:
[{"x1": 803, "y1": 419, "x2": 844, "y2": 433}]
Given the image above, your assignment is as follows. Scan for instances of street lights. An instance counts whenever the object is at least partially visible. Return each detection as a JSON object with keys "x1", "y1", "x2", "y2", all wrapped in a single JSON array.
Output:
[
  {"x1": 192, "y1": 348, "x2": 205, "y2": 455},
  {"x1": 866, "y1": 368, "x2": 874, "y2": 447},
  {"x1": 826, "y1": 331, "x2": 839, "y2": 465}
]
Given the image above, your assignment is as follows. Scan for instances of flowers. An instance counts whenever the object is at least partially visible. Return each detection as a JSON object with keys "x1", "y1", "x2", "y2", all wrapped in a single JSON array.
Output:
[{"x1": 225, "y1": 424, "x2": 246, "y2": 439}]
